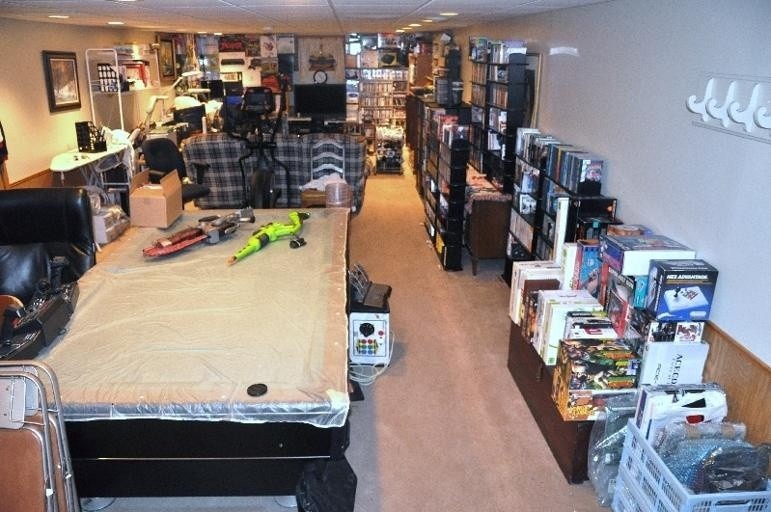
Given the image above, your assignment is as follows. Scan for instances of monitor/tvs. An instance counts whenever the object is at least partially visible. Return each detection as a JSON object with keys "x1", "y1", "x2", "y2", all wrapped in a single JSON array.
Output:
[{"x1": 293, "y1": 84, "x2": 347, "y2": 119}]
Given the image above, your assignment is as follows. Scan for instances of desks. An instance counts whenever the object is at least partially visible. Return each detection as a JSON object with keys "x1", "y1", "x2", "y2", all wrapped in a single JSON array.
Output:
[
  {"x1": 51, "y1": 143, "x2": 128, "y2": 188},
  {"x1": 35, "y1": 208, "x2": 359, "y2": 512},
  {"x1": 185, "y1": 133, "x2": 368, "y2": 212}
]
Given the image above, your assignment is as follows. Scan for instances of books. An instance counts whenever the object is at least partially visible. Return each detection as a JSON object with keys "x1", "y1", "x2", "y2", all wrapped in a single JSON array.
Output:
[
  {"x1": 414, "y1": 36, "x2": 527, "y2": 266},
  {"x1": 507, "y1": 129, "x2": 604, "y2": 265},
  {"x1": 359, "y1": 35, "x2": 414, "y2": 173}
]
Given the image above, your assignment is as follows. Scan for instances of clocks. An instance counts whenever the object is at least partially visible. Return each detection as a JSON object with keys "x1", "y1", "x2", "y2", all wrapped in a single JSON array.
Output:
[{"x1": 313, "y1": 70, "x2": 328, "y2": 84}]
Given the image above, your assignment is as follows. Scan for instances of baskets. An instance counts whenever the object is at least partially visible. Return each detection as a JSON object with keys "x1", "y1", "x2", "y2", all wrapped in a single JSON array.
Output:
[{"x1": 611, "y1": 417, "x2": 771, "y2": 512}]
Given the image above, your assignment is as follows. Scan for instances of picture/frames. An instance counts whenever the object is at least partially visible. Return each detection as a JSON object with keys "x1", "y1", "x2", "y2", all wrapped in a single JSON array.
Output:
[
  {"x1": 157, "y1": 35, "x2": 178, "y2": 82},
  {"x1": 42, "y1": 50, "x2": 82, "y2": 112}
]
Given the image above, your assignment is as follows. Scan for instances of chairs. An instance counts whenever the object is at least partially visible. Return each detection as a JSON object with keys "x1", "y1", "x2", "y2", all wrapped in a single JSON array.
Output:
[
  {"x1": 142, "y1": 138, "x2": 211, "y2": 209},
  {"x1": 0, "y1": 188, "x2": 96, "y2": 307}
]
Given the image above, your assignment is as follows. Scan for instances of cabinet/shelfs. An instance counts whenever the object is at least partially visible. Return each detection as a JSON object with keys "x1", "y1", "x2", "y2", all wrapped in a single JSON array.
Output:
[
  {"x1": 346, "y1": 67, "x2": 409, "y2": 129},
  {"x1": 406, "y1": 62, "x2": 625, "y2": 289}
]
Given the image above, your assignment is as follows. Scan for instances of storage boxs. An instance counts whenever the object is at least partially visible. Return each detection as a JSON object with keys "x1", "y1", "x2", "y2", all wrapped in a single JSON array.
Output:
[{"x1": 129, "y1": 167, "x2": 183, "y2": 228}]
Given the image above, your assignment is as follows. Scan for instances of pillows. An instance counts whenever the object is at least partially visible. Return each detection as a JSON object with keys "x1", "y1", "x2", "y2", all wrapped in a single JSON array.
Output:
[{"x1": 0, "y1": 243, "x2": 46, "y2": 306}]
